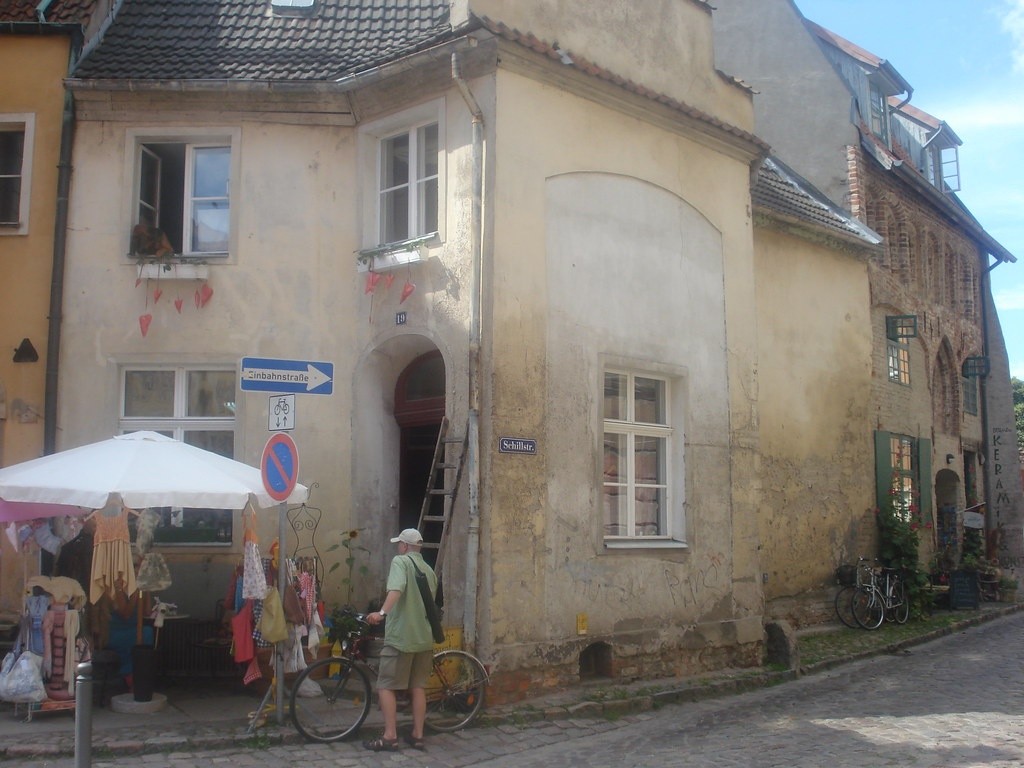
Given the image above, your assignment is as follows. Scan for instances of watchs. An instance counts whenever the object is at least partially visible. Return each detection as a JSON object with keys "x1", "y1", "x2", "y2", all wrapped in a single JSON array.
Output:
[{"x1": 379, "y1": 610, "x2": 388, "y2": 617}]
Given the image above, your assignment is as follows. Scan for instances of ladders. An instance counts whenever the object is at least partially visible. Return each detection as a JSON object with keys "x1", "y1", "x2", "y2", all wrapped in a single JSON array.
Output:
[{"x1": 418, "y1": 416, "x2": 470, "y2": 578}]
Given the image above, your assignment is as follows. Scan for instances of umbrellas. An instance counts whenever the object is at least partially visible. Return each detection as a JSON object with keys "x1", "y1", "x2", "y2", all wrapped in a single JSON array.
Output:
[{"x1": 1, "y1": 430, "x2": 309, "y2": 701}]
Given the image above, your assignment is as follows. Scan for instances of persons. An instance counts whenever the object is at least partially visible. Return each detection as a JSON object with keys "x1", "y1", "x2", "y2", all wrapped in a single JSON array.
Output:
[
  {"x1": 98, "y1": 561, "x2": 154, "y2": 691},
  {"x1": 132, "y1": 223, "x2": 173, "y2": 257},
  {"x1": 363, "y1": 529, "x2": 439, "y2": 750}
]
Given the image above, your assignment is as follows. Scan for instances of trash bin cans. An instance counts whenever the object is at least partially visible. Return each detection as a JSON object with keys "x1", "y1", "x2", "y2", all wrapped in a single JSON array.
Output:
[{"x1": 369, "y1": 598, "x2": 387, "y2": 638}]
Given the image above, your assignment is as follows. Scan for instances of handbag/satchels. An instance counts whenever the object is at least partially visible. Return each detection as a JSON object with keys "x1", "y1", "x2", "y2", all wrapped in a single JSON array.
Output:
[{"x1": 0, "y1": 629, "x2": 48, "y2": 703}]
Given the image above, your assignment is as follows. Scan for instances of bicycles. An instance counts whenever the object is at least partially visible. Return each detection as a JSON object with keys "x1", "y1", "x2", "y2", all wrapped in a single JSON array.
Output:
[
  {"x1": 834, "y1": 555, "x2": 910, "y2": 632},
  {"x1": 289, "y1": 608, "x2": 489, "y2": 745}
]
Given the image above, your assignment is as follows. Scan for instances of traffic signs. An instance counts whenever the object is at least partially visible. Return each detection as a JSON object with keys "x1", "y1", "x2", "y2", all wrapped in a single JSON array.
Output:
[{"x1": 240, "y1": 356, "x2": 333, "y2": 396}]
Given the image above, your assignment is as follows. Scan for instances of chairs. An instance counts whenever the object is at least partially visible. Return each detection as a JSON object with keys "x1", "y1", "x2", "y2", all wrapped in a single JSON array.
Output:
[{"x1": 193, "y1": 599, "x2": 232, "y2": 681}]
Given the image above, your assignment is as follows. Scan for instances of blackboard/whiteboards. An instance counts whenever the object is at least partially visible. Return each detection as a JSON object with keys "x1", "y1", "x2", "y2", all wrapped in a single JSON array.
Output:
[{"x1": 949, "y1": 569, "x2": 980, "y2": 611}]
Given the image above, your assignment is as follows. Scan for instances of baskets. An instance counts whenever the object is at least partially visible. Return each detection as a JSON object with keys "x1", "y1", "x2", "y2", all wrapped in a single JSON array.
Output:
[{"x1": 836, "y1": 565, "x2": 857, "y2": 585}]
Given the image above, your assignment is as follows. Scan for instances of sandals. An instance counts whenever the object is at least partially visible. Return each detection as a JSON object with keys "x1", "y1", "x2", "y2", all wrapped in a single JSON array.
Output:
[
  {"x1": 405, "y1": 733, "x2": 424, "y2": 749},
  {"x1": 363, "y1": 736, "x2": 399, "y2": 751}
]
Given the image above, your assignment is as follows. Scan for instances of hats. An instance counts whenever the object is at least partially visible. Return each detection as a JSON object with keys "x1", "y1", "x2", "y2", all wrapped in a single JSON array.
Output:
[{"x1": 390, "y1": 529, "x2": 423, "y2": 546}]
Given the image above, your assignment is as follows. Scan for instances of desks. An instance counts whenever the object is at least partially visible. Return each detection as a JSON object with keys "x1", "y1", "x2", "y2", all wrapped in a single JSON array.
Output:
[{"x1": 141, "y1": 612, "x2": 192, "y2": 650}]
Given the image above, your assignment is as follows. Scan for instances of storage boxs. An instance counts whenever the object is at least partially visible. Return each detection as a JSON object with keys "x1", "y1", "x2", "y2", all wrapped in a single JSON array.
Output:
[{"x1": 251, "y1": 642, "x2": 334, "y2": 696}]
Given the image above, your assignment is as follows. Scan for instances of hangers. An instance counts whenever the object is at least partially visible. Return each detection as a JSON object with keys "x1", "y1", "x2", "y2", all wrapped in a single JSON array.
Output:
[{"x1": 83, "y1": 492, "x2": 142, "y2": 521}]
